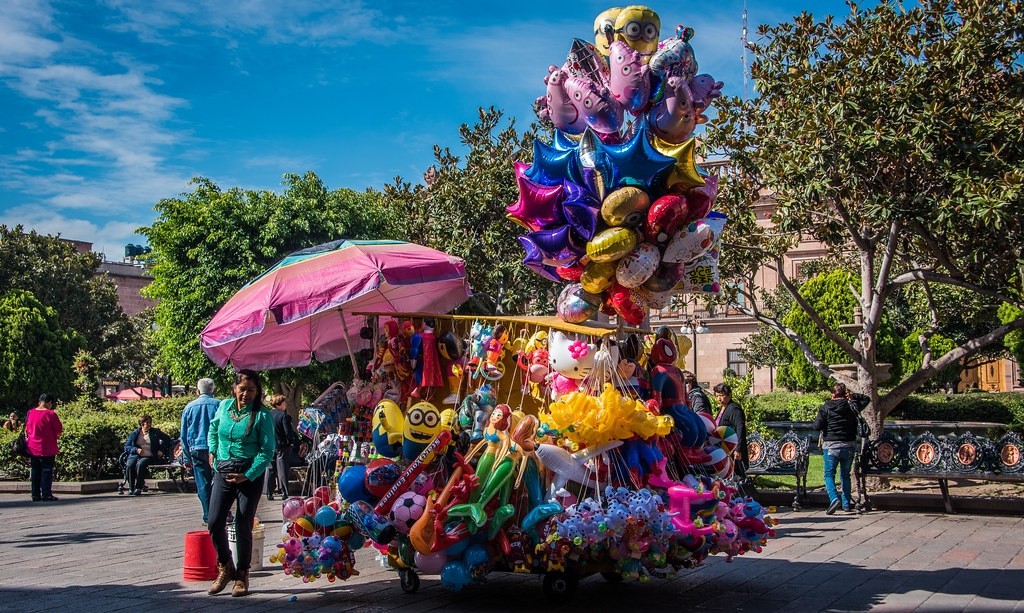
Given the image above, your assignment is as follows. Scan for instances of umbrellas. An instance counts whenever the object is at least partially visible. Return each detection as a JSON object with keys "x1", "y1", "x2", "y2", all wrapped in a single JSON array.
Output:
[{"x1": 200, "y1": 240, "x2": 473, "y2": 379}]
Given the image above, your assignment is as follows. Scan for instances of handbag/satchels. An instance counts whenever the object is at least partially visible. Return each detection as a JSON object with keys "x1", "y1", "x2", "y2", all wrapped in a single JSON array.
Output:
[
  {"x1": 215, "y1": 458, "x2": 249, "y2": 481},
  {"x1": 846, "y1": 400, "x2": 872, "y2": 438},
  {"x1": 11, "y1": 432, "x2": 31, "y2": 458}
]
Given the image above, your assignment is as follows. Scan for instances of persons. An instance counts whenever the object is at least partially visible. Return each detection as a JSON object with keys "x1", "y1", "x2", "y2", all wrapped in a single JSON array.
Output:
[
  {"x1": 207, "y1": 369, "x2": 276, "y2": 597},
  {"x1": 713, "y1": 383, "x2": 750, "y2": 474},
  {"x1": 26, "y1": 393, "x2": 63, "y2": 502},
  {"x1": 124, "y1": 414, "x2": 171, "y2": 495},
  {"x1": 813, "y1": 383, "x2": 871, "y2": 515},
  {"x1": 3, "y1": 411, "x2": 21, "y2": 432},
  {"x1": 265, "y1": 394, "x2": 296, "y2": 500},
  {"x1": 682, "y1": 370, "x2": 712, "y2": 415},
  {"x1": 181, "y1": 378, "x2": 234, "y2": 526}
]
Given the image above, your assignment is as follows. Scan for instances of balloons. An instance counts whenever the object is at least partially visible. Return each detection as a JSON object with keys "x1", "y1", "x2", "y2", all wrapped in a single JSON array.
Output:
[
  {"x1": 505, "y1": 4, "x2": 725, "y2": 324},
  {"x1": 270, "y1": 318, "x2": 780, "y2": 594}
]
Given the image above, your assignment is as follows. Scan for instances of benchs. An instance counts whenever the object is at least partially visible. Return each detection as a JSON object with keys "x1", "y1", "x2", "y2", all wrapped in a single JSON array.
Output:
[
  {"x1": 744, "y1": 432, "x2": 811, "y2": 512},
  {"x1": 119, "y1": 440, "x2": 190, "y2": 494},
  {"x1": 853, "y1": 431, "x2": 1024, "y2": 514}
]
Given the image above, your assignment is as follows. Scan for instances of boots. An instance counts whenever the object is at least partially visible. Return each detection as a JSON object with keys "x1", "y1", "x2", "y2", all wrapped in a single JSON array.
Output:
[
  {"x1": 208, "y1": 557, "x2": 237, "y2": 595},
  {"x1": 231, "y1": 565, "x2": 250, "y2": 597}
]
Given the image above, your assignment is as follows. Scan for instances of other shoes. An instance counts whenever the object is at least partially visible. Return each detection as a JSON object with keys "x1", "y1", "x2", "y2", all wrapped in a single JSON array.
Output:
[
  {"x1": 227, "y1": 514, "x2": 235, "y2": 522},
  {"x1": 41, "y1": 495, "x2": 60, "y2": 501},
  {"x1": 201, "y1": 521, "x2": 209, "y2": 527},
  {"x1": 133, "y1": 489, "x2": 142, "y2": 495},
  {"x1": 267, "y1": 494, "x2": 275, "y2": 500},
  {"x1": 865, "y1": 505, "x2": 873, "y2": 512},
  {"x1": 855, "y1": 505, "x2": 862, "y2": 515},
  {"x1": 281, "y1": 494, "x2": 288, "y2": 500},
  {"x1": 31, "y1": 496, "x2": 41, "y2": 502},
  {"x1": 825, "y1": 498, "x2": 842, "y2": 515},
  {"x1": 844, "y1": 507, "x2": 853, "y2": 512},
  {"x1": 127, "y1": 489, "x2": 133, "y2": 495}
]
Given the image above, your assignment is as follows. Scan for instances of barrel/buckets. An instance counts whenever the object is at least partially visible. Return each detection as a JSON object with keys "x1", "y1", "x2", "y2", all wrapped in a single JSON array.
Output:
[
  {"x1": 182, "y1": 531, "x2": 219, "y2": 581},
  {"x1": 226, "y1": 524, "x2": 266, "y2": 571}
]
